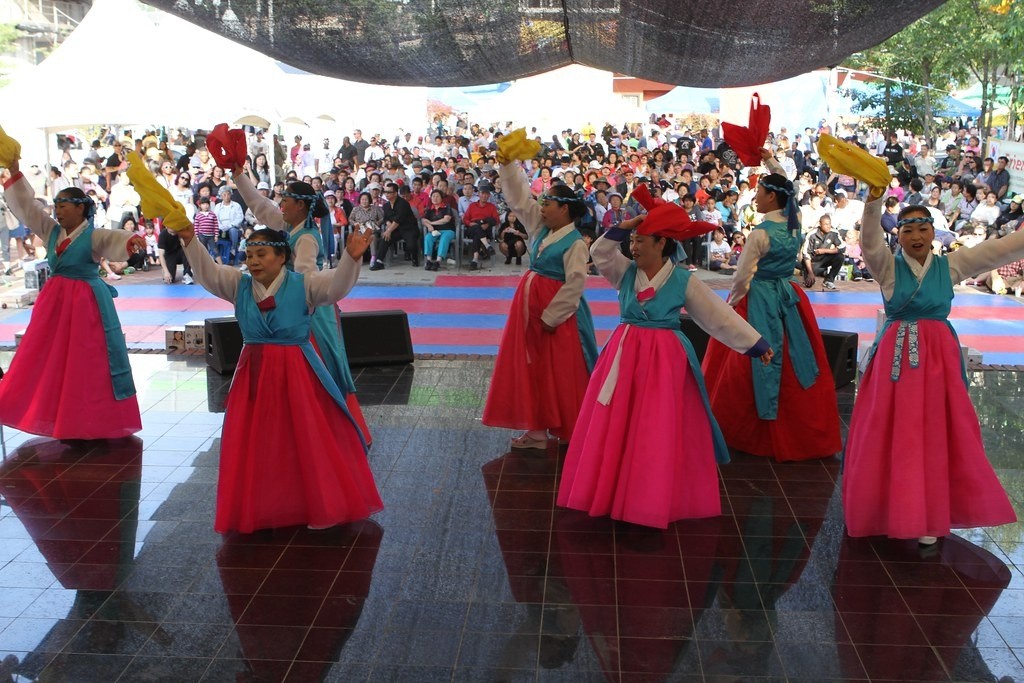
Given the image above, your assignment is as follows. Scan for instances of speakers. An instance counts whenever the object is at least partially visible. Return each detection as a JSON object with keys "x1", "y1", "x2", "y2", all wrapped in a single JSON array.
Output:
[
  {"x1": 820, "y1": 329, "x2": 858, "y2": 389},
  {"x1": 205, "y1": 317, "x2": 243, "y2": 374},
  {"x1": 339, "y1": 309, "x2": 414, "y2": 369},
  {"x1": 679, "y1": 314, "x2": 711, "y2": 367}
]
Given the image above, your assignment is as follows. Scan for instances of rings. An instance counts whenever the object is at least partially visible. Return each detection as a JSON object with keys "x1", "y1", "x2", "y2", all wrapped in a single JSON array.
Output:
[{"x1": 364, "y1": 238, "x2": 367, "y2": 241}]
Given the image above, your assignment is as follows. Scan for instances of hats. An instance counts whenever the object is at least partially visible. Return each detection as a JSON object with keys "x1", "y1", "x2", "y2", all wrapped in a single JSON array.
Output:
[
  {"x1": 324, "y1": 189, "x2": 336, "y2": 198},
  {"x1": 1011, "y1": 194, "x2": 1022, "y2": 204},
  {"x1": 623, "y1": 168, "x2": 633, "y2": 173},
  {"x1": 594, "y1": 177, "x2": 611, "y2": 190},
  {"x1": 257, "y1": 181, "x2": 269, "y2": 190},
  {"x1": 477, "y1": 165, "x2": 493, "y2": 193},
  {"x1": 113, "y1": 141, "x2": 121, "y2": 146}
]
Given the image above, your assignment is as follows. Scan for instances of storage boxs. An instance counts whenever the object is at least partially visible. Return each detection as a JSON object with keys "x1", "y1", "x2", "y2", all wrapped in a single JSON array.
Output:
[
  {"x1": 858, "y1": 308, "x2": 983, "y2": 384},
  {"x1": 185, "y1": 320, "x2": 206, "y2": 350},
  {"x1": 165, "y1": 327, "x2": 185, "y2": 350}
]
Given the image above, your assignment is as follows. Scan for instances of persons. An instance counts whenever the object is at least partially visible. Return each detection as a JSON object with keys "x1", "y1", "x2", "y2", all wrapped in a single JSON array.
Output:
[
  {"x1": 844, "y1": 185, "x2": 1024, "y2": 546},
  {"x1": 701, "y1": 148, "x2": 843, "y2": 460},
  {"x1": 229, "y1": 160, "x2": 371, "y2": 444},
  {"x1": 482, "y1": 146, "x2": 600, "y2": 448},
  {"x1": 0, "y1": 153, "x2": 148, "y2": 441},
  {"x1": 557, "y1": 214, "x2": 772, "y2": 527},
  {"x1": 176, "y1": 224, "x2": 385, "y2": 532},
  {"x1": 0, "y1": 113, "x2": 1024, "y2": 295}
]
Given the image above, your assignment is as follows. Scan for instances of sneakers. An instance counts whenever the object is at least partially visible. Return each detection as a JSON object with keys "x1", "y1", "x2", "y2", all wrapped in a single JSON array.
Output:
[
  {"x1": 822, "y1": 280, "x2": 836, "y2": 289},
  {"x1": 181, "y1": 273, "x2": 194, "y2": 285}
]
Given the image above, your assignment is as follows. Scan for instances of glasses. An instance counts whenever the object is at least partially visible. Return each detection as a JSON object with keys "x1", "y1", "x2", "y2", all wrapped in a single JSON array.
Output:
[
  {"x1": 115, "y1": 144, "x2": 121, "y2": 147},
  {"x1": 383, "y1": 191, "x2": 394, "y2": 193},
  {"x1": 162, "y1": 166, "x2": 173, "y2": 169},
  {"x1": 179, "y1": 176, "x2": 190, "y2": 182}
]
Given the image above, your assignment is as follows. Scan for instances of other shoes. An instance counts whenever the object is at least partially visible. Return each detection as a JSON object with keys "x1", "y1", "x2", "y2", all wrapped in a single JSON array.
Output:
[
  {"x1": 853, "y1": 272, "x2": 874, "y2": 282},
  {"x1": 425, "y1": 260, "x2": 440, "y2": 270},
  {"x1": 505, "y1": 256, "x2": 521, "y2": 265},
  {"x1": 412, "y1": 255, "x2": 419, "y2": 266},
  {"x1": 470, "y1": 262, "x2": 477, "y2": 269},
  {"x1": 369, "y1": 261, "x2": 384, "y2": 270},
  {"x1": 511, "y1": 432, "x2": 548, "y2": 449},
  {"x1": 918, "y1": 535, "x2": 937, "y2": 545},
  {"x1": 369, "y1": 255, "x2": 377, "y2": 267},
  {"x1": 486, "y1": 246, "x2": 495, "y2": 256}
]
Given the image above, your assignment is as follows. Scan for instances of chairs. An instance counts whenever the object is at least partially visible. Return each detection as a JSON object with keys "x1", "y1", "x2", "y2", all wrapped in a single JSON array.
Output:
[{"x1": 339, "y1": 207, "x2": 491, "y2": 270}]
